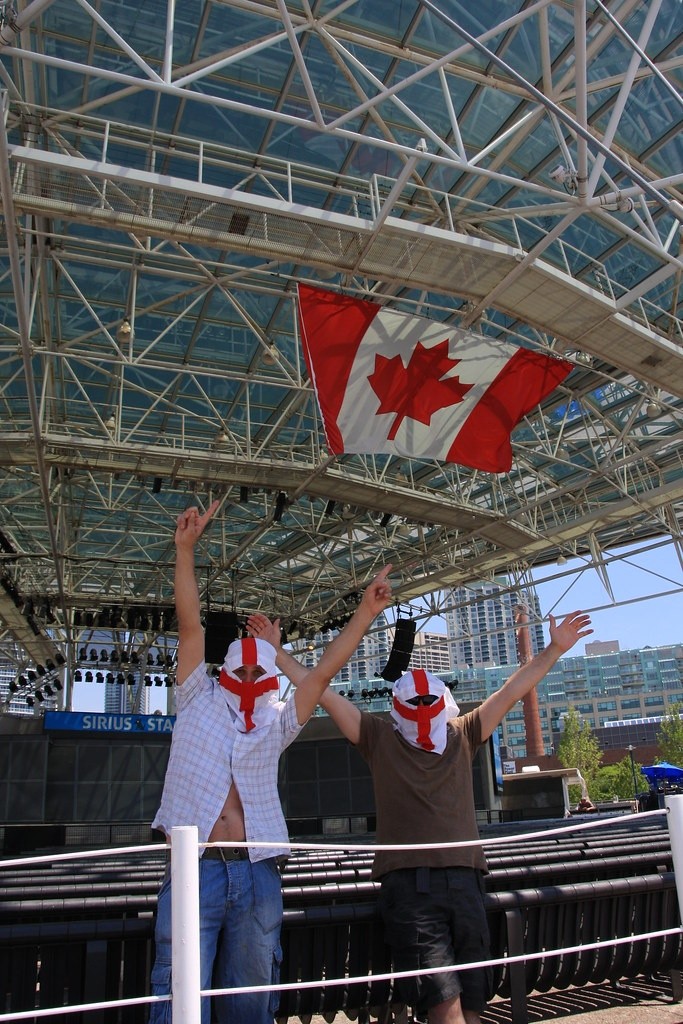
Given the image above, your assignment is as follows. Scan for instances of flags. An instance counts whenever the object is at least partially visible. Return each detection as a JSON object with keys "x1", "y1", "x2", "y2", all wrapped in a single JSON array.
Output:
[{"x1": 296, "y1": 280, "x2": 577, "y2": 473}]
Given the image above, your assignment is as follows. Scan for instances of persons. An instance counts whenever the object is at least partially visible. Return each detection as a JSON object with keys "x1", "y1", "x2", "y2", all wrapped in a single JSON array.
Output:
[
  {"x1": 578, "y1": 797, "x2": 596, "y2": 811},
  {"x1": 245, "y1": 610, "x2": 594, "y2": 1024},
  {"x1": 149, "y1": 499, "x2": 392, "y2": 1024},
  {"x1": 645, "y1": 788, "x2": 659, "y2": 811}
]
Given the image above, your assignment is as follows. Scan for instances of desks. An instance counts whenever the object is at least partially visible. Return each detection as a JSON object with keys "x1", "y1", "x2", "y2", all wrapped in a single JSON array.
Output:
[{"x1": 501, "y1": 767, "x2": 582, "y2": 822}]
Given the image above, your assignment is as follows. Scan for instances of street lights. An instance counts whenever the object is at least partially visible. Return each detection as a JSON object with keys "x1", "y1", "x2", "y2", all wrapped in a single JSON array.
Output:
[{"x1": 625, "y1": 745, "x2": 638, "y2": 794}]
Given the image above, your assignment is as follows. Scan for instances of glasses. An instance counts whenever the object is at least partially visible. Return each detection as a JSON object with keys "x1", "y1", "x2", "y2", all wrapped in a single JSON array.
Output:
[{"x1": 406, "y1": 695, "x2": 439, "y2": 705}]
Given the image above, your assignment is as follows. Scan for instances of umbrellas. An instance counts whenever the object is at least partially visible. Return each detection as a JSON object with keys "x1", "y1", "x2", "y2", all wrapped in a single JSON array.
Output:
[{"x1": 641, "y1": 761, "x2": 683, "y2": 795}]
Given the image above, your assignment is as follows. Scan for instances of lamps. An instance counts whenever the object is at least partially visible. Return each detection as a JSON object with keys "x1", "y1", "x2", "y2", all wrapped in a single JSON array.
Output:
[
  {"x1": 556, "y1": 443, "x2": 569, "y2": 459},
  {"x1": 74, "y1": 647, "x2": 178, "y2": 687},
  {"x1": 215, "y1": 428, "x2": 229, "y2": 443},
  {"x1": 8, "y1": 652, "x2": 65, "y2": 708},
  {"x1": 647, "y1": 398, "x2": 662, "y2": 418},
  {"x1": 339, "y1": 679, "x2": 458, "y2": 702},
  {"x1": 262, "y1": 341, "x2": 279, "y2": 366},
  {"x1": 0, "y1": 533, "x2": 179, "y2": 636},
  {"x1": 201, "y1": 588, "x2": 367, "y2": 644},
  {"x1": 324, "y1": 500, "x2": 336, "y2": 518},
  {"x1": 106, "y1": 412, "x2": 115, "y2": 430},
  {"x1": 273, "y1": 490, "x2": 285, "y2": 521},
  {"x1": 117, "y1": 319, "x2": 135, "y2": 343}
]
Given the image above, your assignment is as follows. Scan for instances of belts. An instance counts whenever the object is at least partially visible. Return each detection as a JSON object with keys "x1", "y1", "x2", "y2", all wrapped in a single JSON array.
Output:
[{"x1": 166, "y1": 847, "x2": 246, "y2": 864}]
